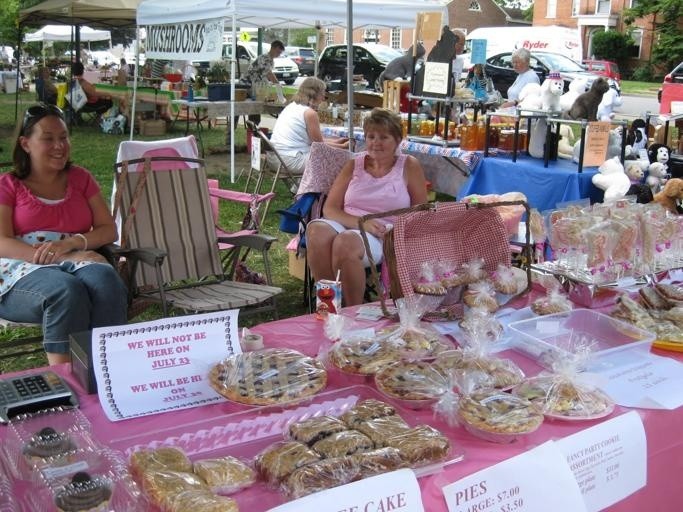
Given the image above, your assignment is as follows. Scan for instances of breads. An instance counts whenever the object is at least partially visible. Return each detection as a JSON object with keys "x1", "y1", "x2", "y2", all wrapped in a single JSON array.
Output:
[
  {"x1": 461, "y1": 269, "x2": 486, "y2": 285},
  {"x1": 375, "y1": 356, "x2": 456, "y2": 408},
  {"x1": 132, "y1": 447, "x2": 193, "y2": 483},
  {"x1": 385, "y1": 424, "x2": 450, "y2": 466},
  {"x1": 354, "y1": 412, "x2": 412, "y2": 448},
  {"x1": 517, "y1": 374, "x2": 609, "y2": 420},
  {"x1": 457, "y1": 388, "x2": 544, "y2": 442},
  {"x1": 458, "y1": 316, "x2": 503, "y2": 342},
  {"x1": 444, "y1": 351, "x2": 524, "y2": 392},
  {"x1": 341, "y1": 398, "x2": 396, "y2": 429},
  {"x1": 143, "y1": 470, "x2": 209, "y2": 508},
  {"x1": 288, "y1": 456, "x2": 363, "y2": 499},
  {"x1": 165, "y1": 489, "x2": 238, "y2": 512},
  {"x1": 312, "y1": 429, "x2": 375, "y2": 458},
  {"x1": 208, "y1": 348, "x2": 328, "y2": 407},
  {"x1": 195, "y1": 455, "x2": 257, "y2": 496},
  {"x1": 463, "y1": 293, "x2": 498, "y2": 313},
  {"x1": 329, "y1": 339, "x2": 404, "y2": 378},
  {"x1": 353, "y1": 446, "x2": 409, "y2": 476},
  {"x1": 288, "y1": 415, "x2": 348, "y2": 448},
  {"x1": 255, "y1": 441, "x2": 321, "y2": 487},
  {"x1": 492, "y1": 270, "x2": 522, "y2": 294},
  {"x1": 531, "y1": 297, "x2": 574, "y2": 317},
  {"x1": 442, "y1": 274, "x2": 462, "y2": 289},
  {"x1": 376, "y1": 321, "x2": 440, "y2": 360},
  {"x1": 413, "y1": 273, "x2": 447, "y2": 296}
]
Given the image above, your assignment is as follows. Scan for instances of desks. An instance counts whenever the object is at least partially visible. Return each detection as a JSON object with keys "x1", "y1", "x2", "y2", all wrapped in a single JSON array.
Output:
[
  {"x1": 0, "y1": 259, "x2": 682, "y2": 510},
  {"x1": 348, "y1": 85, "x2": 629, "y2": 236},
  {"x1": 32, "y1": 51, "x2": 350, "y2": 163}
]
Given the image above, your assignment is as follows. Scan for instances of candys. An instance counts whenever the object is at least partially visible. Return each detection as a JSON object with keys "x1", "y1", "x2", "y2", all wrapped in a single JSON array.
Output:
[{"x1": 551, "y1": 202, "x2": 683, "y2": 282}]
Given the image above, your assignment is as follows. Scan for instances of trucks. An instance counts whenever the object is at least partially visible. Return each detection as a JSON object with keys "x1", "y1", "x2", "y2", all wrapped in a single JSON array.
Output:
[{"x1": 456, "y1": 24, "x2": 585, "y2": 85}]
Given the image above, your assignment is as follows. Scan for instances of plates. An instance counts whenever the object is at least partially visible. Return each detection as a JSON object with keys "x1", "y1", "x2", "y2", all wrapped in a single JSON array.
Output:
[
  {"x1": 377, "y1": 363, "x2": 447, "y2": 410},
  {"x1": 458, "y1": 419, "x2": 541, "y2": 441},
  {"x1": 511, "y1": 386, "x2": 615, "y2": 422},
  {"x1": 491, "y1": 383, "x2": 521, "y2": 392},
  {"x1": 608, "y1": 310, "x2": 682, "y2": 354},
  {"x1": 336, "y1": 367, "x2": 376, "y2": 384},
  {"x1": 419, "y1": 354, "x2": 437, "y2": 361}
]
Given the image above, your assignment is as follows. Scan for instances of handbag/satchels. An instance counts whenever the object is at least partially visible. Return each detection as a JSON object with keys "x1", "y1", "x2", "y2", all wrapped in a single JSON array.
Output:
[
  {"x1": 65, "y1": 85, "x2": 88, "y2": 113},
  {"x1": 101, "y1": 114, "x2": 127, "y2": 136}
]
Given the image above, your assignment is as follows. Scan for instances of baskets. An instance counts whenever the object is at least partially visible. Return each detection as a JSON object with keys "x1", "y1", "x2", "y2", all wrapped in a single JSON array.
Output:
[{"x1": 382, "y1": 206, "x2": 514, "y2": 309}]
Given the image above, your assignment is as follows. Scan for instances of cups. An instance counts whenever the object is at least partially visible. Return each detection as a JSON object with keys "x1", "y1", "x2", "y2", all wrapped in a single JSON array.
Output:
[
  {"x1": 255, "y1": 81, "x2": 271, "y2": 101},
  {"x1": 518, "y1": 222, "x2": 531, "y2": 241},
  {"x1": 239, "y1": 334, "x2": 263, "y2": 351}
]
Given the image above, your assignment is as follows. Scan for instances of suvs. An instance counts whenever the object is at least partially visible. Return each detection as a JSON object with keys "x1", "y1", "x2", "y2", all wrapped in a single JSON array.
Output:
[{"x1": 579, "y1": 57, "x2": 622, "y2": 86}]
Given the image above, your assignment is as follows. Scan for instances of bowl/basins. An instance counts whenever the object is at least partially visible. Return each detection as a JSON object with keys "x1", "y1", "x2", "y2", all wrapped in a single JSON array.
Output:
[{"x1": 162, "y1": 75, "x2": 181, "y2": 82}]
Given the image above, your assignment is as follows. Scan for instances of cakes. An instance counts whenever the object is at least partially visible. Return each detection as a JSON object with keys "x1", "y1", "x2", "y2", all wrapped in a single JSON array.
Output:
[
  {"x1": 22, "y1": 427, "x2": 76, "y2": 470},
  {"x1": 52, "y1": 471, "x2": 112, "y2": 512}
]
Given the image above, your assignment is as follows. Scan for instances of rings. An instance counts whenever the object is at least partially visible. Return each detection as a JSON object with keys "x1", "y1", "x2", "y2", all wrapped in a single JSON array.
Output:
[{"x1": 48, "y1": 252, "x2": 54, "y2": 256}]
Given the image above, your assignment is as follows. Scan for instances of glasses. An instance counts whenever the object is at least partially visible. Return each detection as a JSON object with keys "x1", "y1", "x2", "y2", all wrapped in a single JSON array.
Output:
[{"x1": 22, "y1": 103, "x2": 65, "y2": 132}]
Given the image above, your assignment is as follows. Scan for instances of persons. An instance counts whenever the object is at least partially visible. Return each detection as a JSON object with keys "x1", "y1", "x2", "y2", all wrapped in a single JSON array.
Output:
[
  {"x1": 452, "y1": 30, "x2": 465, "y2": 73},
  {"x1": 0, "y1": 105, "x2": 129, "y2": 366},
  {"x1": 501, "y1": 48, "x2": 540, "y2": 108},
  {"x1": 266, "y1": 79, "x2": 355, "y2": 194},
  {"x1": 305, "y1": 107, "x2": 428, "y2": 307},
  {"x1": 0, "y1": 43, "x2": 193, "y2": 126},
  {"x1": 226, "y1": 40, "x2": 284, "y2": 145}
]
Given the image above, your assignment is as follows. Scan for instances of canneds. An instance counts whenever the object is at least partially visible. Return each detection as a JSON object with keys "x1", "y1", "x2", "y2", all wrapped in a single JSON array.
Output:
[{"x1": 499, "y1": 130, "x2": 528, "y2": 151}]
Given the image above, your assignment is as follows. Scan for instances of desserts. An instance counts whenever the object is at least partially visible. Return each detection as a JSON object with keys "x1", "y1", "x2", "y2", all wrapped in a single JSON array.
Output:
[{"x1": 607, "y1": 283, "x2": 683, "y2": 344}]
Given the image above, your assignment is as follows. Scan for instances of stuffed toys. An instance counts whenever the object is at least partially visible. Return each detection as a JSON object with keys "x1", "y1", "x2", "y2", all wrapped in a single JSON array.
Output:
[
  {"x1": 519, "y1": 70, "x2": 683, "y2": 215},
  {"x1": 379, "y1": 42, "x2": 425, "y2": 90}
]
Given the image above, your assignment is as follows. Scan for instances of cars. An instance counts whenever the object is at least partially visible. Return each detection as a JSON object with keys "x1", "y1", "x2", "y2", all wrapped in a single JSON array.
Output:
[
  {"x1": 313, "y1": 42, "x2": 423, "y2": 95},
  {"x1": 462, "y1": 49, "x2": 622, "y2": 100},
  {"x1": 67, "y1": 39, "x2": 318, "y2": 85},
  {"x1": 652, "y1": 61, "x2": 682, "y2": 104}
]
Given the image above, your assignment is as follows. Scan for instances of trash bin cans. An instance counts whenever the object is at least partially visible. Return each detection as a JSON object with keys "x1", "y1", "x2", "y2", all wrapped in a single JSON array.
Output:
[{"x1": 2, "y1": 73, "x2": 20, "y2": 93}]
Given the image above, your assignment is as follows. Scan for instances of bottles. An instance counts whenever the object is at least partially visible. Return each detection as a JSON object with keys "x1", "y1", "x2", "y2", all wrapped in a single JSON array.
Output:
[
  {"x1": 195, "y1": 75, "x2": 208, "y2": 96},
  {"x1": 400, "y1": 114, "x2": 527, "y2": 152},
  {"x1": 187, "y1": 85, "x2": 193, "y2": 102}
]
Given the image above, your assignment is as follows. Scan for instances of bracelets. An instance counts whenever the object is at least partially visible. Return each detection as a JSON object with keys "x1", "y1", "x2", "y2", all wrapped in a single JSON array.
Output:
[
  {"x1": 514, "y1": 100, "x2": 518, "y2": 106},
  {"x1": 75, "y1": 234, "x2": 88, "y2": 252}
]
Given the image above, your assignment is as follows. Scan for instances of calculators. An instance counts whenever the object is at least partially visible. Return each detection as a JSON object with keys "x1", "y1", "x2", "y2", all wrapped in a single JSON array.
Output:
[{"x1": 0, "y1": 370, "x2": 80, "y2": 424}]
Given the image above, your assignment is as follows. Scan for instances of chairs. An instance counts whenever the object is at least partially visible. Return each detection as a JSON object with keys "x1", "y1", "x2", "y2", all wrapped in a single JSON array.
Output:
[
  {"x1": 243, "y1": 117, "x2": 301, "y2": 230},
  {"x1": 111, "y1": 135, "x2": 275, "y2": 283},
  {"x1": 104, "y1": 157, "x2": 286, "y2": 323}
]
